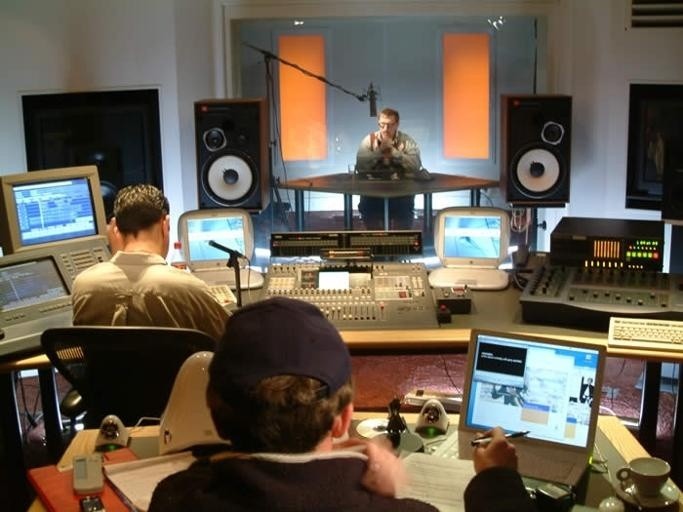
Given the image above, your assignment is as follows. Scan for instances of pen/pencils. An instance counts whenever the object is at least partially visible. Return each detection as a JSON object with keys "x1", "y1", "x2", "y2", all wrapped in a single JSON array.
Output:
[{"x1": 471, "y1": 430, "x2": 531, "y2": 446}]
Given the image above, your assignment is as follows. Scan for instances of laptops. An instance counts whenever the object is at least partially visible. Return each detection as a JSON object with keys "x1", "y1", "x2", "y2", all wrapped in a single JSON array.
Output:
[
  {"x1": 433, "y1": 328, "x2": 606, "y2": 485},
  {"x1": 429, "y1": 208, "x2": 508, "y2": 294},
  {"x1": 178, "y1": 209, "x2": 260, "y2": 292}
]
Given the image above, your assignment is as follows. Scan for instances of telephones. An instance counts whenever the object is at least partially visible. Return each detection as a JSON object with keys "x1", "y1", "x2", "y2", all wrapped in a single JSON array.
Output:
[{"x1": 512, "y1": 244, "x2": 549, "y2": 273}]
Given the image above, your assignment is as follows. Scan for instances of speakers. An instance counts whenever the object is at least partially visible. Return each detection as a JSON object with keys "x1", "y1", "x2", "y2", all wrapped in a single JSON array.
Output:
[
  {"x1": 499, "y1": 92, "x2": 573, "y2": 203},
  {"x1": 196, "y1": 97, "x2": 270, "y2": 208}
]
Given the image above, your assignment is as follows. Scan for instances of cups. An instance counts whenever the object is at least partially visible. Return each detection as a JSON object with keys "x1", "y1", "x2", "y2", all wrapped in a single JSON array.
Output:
[{"x1": 615, "y1": 457, "x2": 671, "y2": 500}]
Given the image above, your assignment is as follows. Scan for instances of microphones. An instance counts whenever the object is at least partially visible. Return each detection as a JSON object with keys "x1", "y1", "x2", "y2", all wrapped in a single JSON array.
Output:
[
  {"x1": 208, "y1": 235, "x2": 246, "y2": 263},
  {"x1": 368, "y1": 84, "x2": 378, "y2": 118}
]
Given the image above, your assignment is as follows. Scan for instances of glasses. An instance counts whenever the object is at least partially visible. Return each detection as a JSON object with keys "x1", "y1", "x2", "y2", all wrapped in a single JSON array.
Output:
[{"x1": 378, "y1": 122, "x2": 396, "y2": 128}]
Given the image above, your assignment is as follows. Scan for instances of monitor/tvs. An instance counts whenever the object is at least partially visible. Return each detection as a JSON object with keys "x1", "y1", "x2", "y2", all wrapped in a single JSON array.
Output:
[
  {"x1": 0, "y1": 255, "x2": 71, "y2": 309},
  {"x1": 0, "y1": 165, "x2": 105, "y2": 254}
]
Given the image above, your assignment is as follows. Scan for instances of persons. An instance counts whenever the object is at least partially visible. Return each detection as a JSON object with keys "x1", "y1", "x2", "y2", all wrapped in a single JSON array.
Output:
[
  {"x1": 68, "y1": 181, "x2": 235, "y2": 354},
  {"x1": 353, "y1": 108, "x2": 423, "y2": 235},
  {"x1": 144, "y1": 293, "x2": 535, "y2": 511},
  {"x1": 489, "y1": 382, "x2": 529, "y2": 407},
  {"x1": 579, "y1": 375, "x2": 594, "y2": 404}
]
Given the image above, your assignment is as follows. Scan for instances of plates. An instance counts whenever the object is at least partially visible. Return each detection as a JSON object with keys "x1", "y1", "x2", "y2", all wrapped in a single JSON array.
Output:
[{"x1": 611, "y1": 477, "x2": 680, "y2": 508}]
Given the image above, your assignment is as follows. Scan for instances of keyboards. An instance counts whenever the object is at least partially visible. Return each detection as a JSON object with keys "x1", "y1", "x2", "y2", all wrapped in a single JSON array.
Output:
[{"x1": 607, "y1": 316, "x2": 683, "y2": 352}]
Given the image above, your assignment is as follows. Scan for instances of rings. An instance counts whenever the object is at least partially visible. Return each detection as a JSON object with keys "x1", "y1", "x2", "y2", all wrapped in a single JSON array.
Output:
[{"x1": 368, "y1": 462, "x2": 381, "y2": 474}]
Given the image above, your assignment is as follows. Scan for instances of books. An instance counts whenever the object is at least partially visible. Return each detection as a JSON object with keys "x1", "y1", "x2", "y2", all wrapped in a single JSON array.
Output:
[
  {"x1": 101, "y1": 451, "x2": 200, "y2": 511},
  {"x1": 391, "y1": 449, "x2": 479, "y2": 511},
  {"x1": 25, "y1": 447, "x2": 141, "y2": 511}
]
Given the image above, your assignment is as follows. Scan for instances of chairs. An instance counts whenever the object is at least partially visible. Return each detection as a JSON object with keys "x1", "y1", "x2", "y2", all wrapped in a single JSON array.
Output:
[{"x1": 41, "y1": 326, "x2": 218, "y2": 427}]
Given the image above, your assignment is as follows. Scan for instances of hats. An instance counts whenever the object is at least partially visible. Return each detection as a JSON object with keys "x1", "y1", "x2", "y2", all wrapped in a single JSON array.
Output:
[{"x1": 209, "y1": 298, "x2": 352, "y2": 406}]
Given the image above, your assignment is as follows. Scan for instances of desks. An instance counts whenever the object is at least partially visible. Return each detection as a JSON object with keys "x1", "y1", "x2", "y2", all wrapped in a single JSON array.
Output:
[
  {"x1": 274, "y1": 173, "x2": 501, "y2": 232},
  {"x1": 31, "y1": 412, "x2": 682, "y2": 512},
  {"x1": 1, "y1": 262, "x2": 683, "y2": 459}
]
